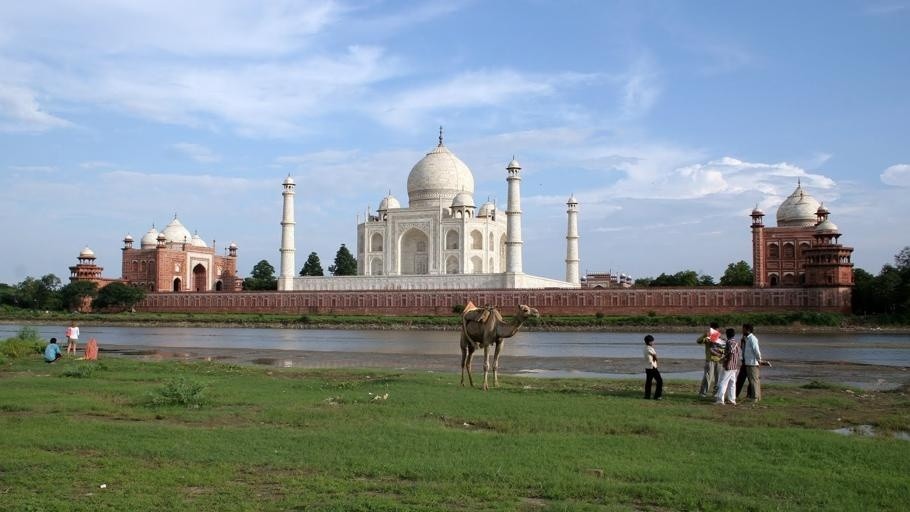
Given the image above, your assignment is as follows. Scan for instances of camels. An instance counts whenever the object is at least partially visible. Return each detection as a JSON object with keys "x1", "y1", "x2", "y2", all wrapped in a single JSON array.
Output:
[{"x1": 460, "y1": 302, "x2": 540, "y2": 393}]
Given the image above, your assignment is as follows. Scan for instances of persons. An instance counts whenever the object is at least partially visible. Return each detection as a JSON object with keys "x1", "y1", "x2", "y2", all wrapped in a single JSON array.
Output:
[
  {"x1": 644, "y1": 335, "x2": 663, "y2": 400},
  {"x1": 736, "y1": 327, "x2": 751, "y2": 398},
  {"x1": 67, "y1": 322, "x2": 79, "y2": 355},
  {"x1": 744, "y1": 324, "x2": 762, "y2": 403},
  {"x1": 712, "y1": 328, "x2": 742, "y2": 405},
  {"x1": 75, "y1": 337, "x2": 98, "y2": 360},
  {"x1": 44, "y1": 338, "x2": 62, "y2": 364},
  {"x1": 697, "y1": 322, "x2": 726, "y2": 397}
]
